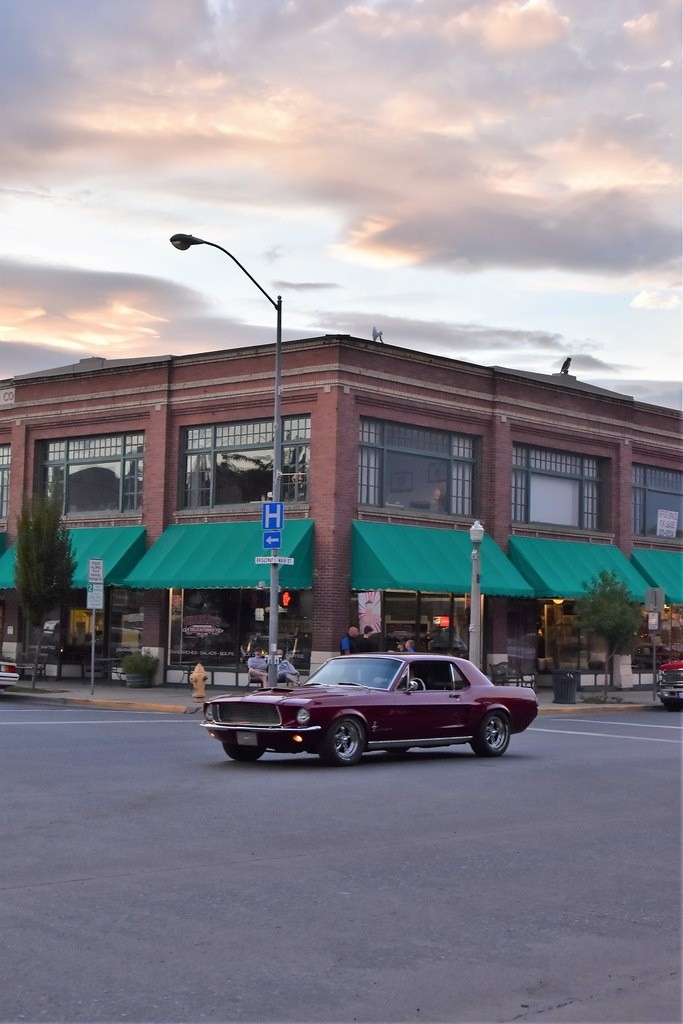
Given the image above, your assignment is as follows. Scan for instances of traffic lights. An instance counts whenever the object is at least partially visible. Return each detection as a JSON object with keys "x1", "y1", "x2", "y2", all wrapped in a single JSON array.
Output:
[{"x1": 277, "y1": 588, "x2": 297, "y2": 609}]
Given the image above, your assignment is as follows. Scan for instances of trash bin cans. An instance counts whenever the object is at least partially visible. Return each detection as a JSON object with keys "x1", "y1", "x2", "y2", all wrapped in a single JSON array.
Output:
[{"x1": 550, "y1": 669, "x2": 581, "y2": 704}]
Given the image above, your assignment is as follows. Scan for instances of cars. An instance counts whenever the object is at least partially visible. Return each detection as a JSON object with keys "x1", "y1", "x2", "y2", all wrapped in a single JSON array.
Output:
[
  {"x1": 656, "y1": 659, "x2": 683, "y2": 713},
  {"x1": 199, "y1": 652, "x2": 538, "y2": 767},
  {"x1": 0, "y1": 660, "x2": 20, "y2": 694}
]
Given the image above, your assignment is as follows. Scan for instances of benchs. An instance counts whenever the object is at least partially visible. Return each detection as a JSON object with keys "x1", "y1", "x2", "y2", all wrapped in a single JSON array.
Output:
[
  {"x1": 247, "y1": 666, "x2": 288, "y2": 689},
  {"x1": 14, "y1": 651, "x2": 49, "y2": 678},
  {"x1": 490, "y1": 662, "x2": 538, "y2": 689}
]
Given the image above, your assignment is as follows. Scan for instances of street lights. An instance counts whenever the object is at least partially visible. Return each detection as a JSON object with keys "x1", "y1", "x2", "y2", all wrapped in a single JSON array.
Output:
[
  {"x1": 167, "y1": 232, "x2": 286, "y2": 698},
  {"x1": 468, "y1": 520, "x2": 486, "y2": 674}
]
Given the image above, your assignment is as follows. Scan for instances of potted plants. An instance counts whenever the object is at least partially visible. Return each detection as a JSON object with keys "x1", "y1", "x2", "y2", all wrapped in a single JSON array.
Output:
[{"x1": 121, "y1": 648, "x2": 160, "y2": 687}]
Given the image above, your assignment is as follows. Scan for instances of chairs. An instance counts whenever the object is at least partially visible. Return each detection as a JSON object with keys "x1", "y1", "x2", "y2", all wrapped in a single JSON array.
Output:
[{"x1": 82, "y1": 655, "x2": 126, "y2": 687}]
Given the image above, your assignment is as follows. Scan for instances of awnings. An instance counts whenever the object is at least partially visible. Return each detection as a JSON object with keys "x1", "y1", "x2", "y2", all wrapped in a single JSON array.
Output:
[
  {"x1": 124, "y1": 518, "x2": 313, "y2": 592},
  {"x1": 508, "y1": 535, "x2": 654, "y2": 605},
  {"x1": 0, "y1": 531, "x2": 8, "y2": 558},
  {"x1": 0, "y1": 525, "x2": 146, "y2": 589},
  {"x1": 630, "y1": 548, "x2": 683, "y2": 606},
  {"x1": 352, "y1": 520, "x2": 534, "y2": 599}
]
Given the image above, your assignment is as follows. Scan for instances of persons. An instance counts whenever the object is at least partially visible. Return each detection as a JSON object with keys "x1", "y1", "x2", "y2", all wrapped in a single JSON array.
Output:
[
  {"x1": 340, "y1": 627, "x2": 359, "y2": 656},
  {"x1": 248, "y1": 649, "x2": 268, "y2": 688},
  {"x1": 357, "y1": 626, "x2": 379, "y2": 653},
  {"x1": 398, "y1": 640, "x2": 415, "y2": 652},
  {"x1": 277, "y1": 650, "x2": 301, "y2": 687}
]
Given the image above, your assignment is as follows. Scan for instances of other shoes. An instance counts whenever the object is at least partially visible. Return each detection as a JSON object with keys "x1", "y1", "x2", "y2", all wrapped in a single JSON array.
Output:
[{"x1": 296, "y1": 680, "x2": 301, "y2": 685}]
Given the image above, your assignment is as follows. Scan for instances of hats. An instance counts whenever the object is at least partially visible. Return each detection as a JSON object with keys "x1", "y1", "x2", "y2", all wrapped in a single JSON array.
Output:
[{"x1": 255, "y1": 647, "x2": 262, "y2": 651}]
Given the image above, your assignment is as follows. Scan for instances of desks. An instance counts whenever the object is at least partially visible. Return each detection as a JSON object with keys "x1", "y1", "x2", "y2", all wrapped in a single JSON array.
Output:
[
  {"x1": 171, "y1": 661, "x2": 210, "y2": 692},
  {"x1": 94, "y1": 658, "x2": 123, "y2": 686}
]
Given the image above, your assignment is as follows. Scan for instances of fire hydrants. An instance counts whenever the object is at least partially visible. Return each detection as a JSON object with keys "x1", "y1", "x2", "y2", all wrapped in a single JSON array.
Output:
[{"x1": 187, "y1": 662, "x2": 208, "y2": 702}]
